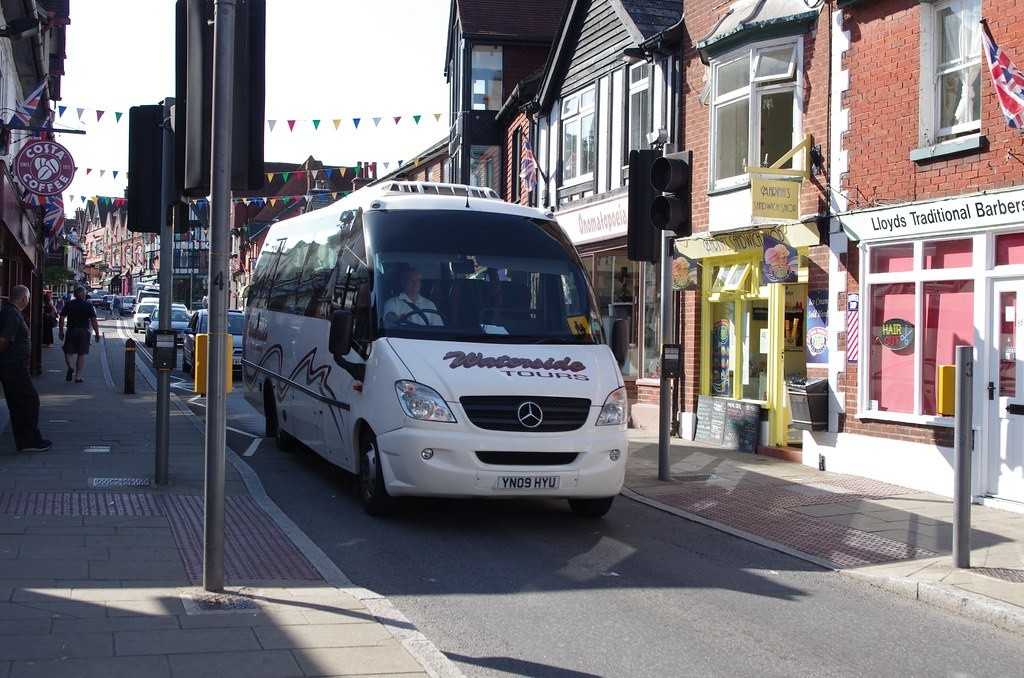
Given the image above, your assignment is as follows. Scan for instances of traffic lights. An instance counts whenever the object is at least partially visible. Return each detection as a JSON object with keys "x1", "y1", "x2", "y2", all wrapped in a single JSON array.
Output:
[{"x1": 649, "y1": 150, "x2": 693, "y2": 239}]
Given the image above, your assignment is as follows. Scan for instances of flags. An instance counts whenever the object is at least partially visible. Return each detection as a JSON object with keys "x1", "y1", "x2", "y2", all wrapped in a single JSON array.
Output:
[
  {"x1": 11, "y1": 86, "x2": 66, "y2": 240},
  {"x1": 521, "y1": 142, "x2": 538, "y2": 192},
  {"x1": 981, "y1": 25, "x2": 1024, "y2": 128}
]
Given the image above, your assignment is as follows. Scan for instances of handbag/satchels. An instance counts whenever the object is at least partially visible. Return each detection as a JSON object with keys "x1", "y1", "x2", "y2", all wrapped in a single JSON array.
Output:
[{"x1": 47, "y1": 312, "x2": 58, "y2": 328}]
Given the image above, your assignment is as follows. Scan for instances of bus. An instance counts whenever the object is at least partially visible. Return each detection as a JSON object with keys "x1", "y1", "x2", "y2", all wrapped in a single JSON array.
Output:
[{"x1": 238, "y1": 182, "x2": 632, "y2": 517}]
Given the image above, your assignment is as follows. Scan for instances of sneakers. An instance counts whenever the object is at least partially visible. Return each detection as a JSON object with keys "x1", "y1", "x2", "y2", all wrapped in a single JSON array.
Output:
[{"x1": 22, "y1": 440, "x2": 53, "y2": 452}]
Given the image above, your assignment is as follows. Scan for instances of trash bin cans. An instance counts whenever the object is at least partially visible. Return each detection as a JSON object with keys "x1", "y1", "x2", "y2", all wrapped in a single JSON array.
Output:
[{"x1": 784, "y1": 375, "x2": 828, "y2": 432}]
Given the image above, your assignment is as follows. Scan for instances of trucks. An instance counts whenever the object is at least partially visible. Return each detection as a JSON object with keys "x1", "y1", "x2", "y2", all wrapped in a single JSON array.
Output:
[{"x1": 137, "y1": 282, "x2": 161, "y2": 290}]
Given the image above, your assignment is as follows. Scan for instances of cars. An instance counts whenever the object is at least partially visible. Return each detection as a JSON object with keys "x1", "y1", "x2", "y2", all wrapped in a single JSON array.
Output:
[
  {"x1": 137, "y1": 290, "x2": 160, "y2": 302},
  {"x1": 133, "y1": 303, "x2": 160, "y2": 333},
  {"x1": 141, "y1": 296, "x2": 160, "y2": 303},
  {"x1": 182, "y1": 309, "x2": 245, "y2": 372},
  {"x1": 171, "y1": 303, "x2": 188, "y2": 309},
  {"x1": 86, "y1": 289, "x2": 136, "y2": 317},
  {"x1": 144, "y1": 307, "x2": 191, "y2": 347}
]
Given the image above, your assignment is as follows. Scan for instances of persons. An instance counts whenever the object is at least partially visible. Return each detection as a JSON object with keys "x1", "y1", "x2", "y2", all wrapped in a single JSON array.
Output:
[
  {"x1": 383, "y1": 268, "x2": 444, "y2": 325},
  {"x1": 59, "y1": 286, "x2": 99, "y2": 382},
  {"x1": 0, "y1": 285, "x2": 53, "y2": 452},
  {"x1": 41, "y1": 294, "x2": 57, "y2": 348},
  {"x1": 85, "y1": 294, "x2": 97, "y2": 344},
  {"x1": 113, "y1": 295, "x2": 120, "y2": 319}
]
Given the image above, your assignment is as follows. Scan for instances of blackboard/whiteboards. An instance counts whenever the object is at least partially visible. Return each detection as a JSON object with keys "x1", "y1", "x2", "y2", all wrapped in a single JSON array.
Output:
[
  {"x1": 723, "y1": 399, "x2": 761, "y2": 455},
  {"x1": 694, "y1": 394, "x2": 727, "y2": 446}
]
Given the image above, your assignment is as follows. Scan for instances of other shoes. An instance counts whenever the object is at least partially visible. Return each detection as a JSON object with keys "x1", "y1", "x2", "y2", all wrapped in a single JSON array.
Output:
[
  {"x1": 66, "y1": 368, "x2": 74, "y2": 382},
  {"x1": 75, "y1": 378, "x2": 83, "y2": 383}
]
